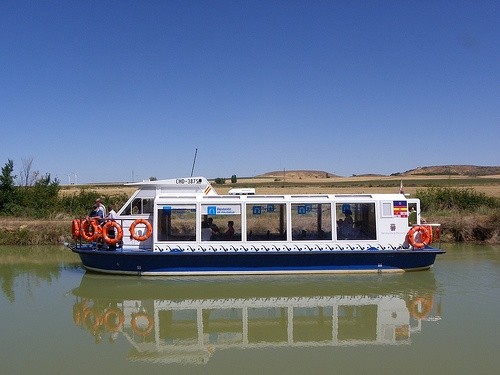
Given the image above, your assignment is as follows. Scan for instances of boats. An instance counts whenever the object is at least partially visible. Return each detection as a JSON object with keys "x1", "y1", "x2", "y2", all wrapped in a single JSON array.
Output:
[
  {"x1": 63, "y1": 267, "x2": 443, "y2": 364},
  {"x1": 62, "y1": 148, "x2": 446, "y2": 276}
]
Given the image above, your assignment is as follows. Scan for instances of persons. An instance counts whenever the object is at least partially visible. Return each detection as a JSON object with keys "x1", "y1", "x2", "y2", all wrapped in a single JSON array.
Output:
[
  {"x1": 201, "y1": 215, "x2": 219, "y2": 241},
  {"x1": 89, "y1": 198, "x2": 106, "y2": 249},
  {"x1": 337, "y1": 209, "x2": 353, "y2": 238},
  {"x1": 408, "y1": 206, "x2": 421, "y2": 222},
  {"x1": 225, "y1": 220, "x2": 234, "y2": 240},
  {"x1": 105, "y1": 205, "x2": 117, "y2": 251}
]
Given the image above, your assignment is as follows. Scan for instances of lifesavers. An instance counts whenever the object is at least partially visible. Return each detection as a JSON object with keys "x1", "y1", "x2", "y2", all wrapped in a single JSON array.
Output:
[
  {"x1": 131, "y1": 313, "x2": 154, "y2": 336},
  {"x1": 72, "y1": 219, "x2": 82, "y2": 240},
  {"x1": 103, "y1": 308, "x2": 125, "y2": 332},
  {"x1": 73, "y1": 303, "x2": 82, "y2": 326},
  {"x1": 129, "y1": 220, "x2": 152, "y2": 241},
  {"x1": 409, "y1": 297, "x2": 431, "y2": 319},
  {"x1": 83, "y1": 308, "x2": 101, "y2": 330},
  {"x1": 80, "y1": 219, "x2": 99, "y2": 242},
  {"x1": 102, "y1": 222, "x2": 123, "y2": 244},
  {"x1": 407, "y1": 225, "x2": 430, "y2": 248},
  {"x1": 427, "y1": 226, "x2": 433, "y2": 246}
]
